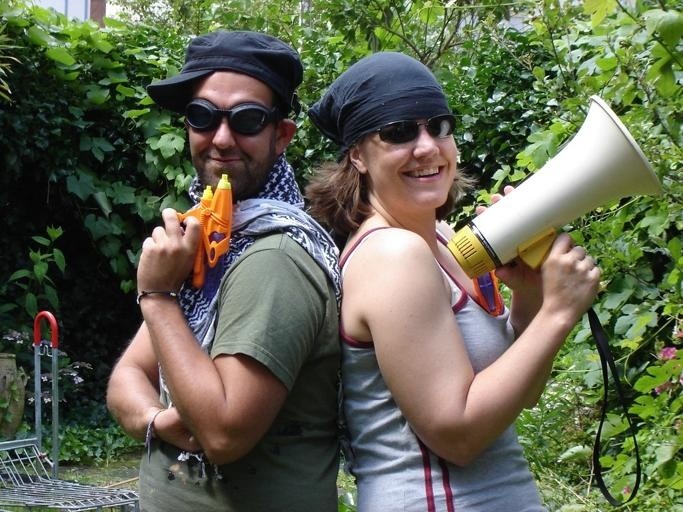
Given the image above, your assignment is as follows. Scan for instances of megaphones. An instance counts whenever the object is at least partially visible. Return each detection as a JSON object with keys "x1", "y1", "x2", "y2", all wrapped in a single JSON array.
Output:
[{"x1": 447, "y1": 94, "x2": 663, "y2": 280}]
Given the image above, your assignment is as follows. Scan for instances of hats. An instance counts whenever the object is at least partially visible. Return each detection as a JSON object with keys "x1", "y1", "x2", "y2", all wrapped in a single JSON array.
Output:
[{"x1": 144, "y1": 28, "x2": 304, "y2": 118}]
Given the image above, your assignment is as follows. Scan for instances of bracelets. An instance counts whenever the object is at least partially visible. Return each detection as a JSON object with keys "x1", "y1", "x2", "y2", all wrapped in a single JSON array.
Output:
[
  {"x1": 145, "y1": 407, "x2": 165, "y2": 441},
  {"x1": 135, "y1": 289, "x2": 179, "y2": 304}
]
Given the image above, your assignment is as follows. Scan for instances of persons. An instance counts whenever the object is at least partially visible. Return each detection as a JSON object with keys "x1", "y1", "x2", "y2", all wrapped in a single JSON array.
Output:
[
  {"x1": 103, "y1": 26, "x2": 341, "y2": 510},
  {"x1": 305, "y1": 50, "x2": 603, "y2": 510}
]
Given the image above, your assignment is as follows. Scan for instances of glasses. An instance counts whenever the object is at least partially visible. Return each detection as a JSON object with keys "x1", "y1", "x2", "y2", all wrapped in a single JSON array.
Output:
[
  {"x1": 182, "y1": 98, "x2": 282, "y2": 136},
  {"x1": 369, "y1": 112, "x2": 458, "y2": 145}
]
